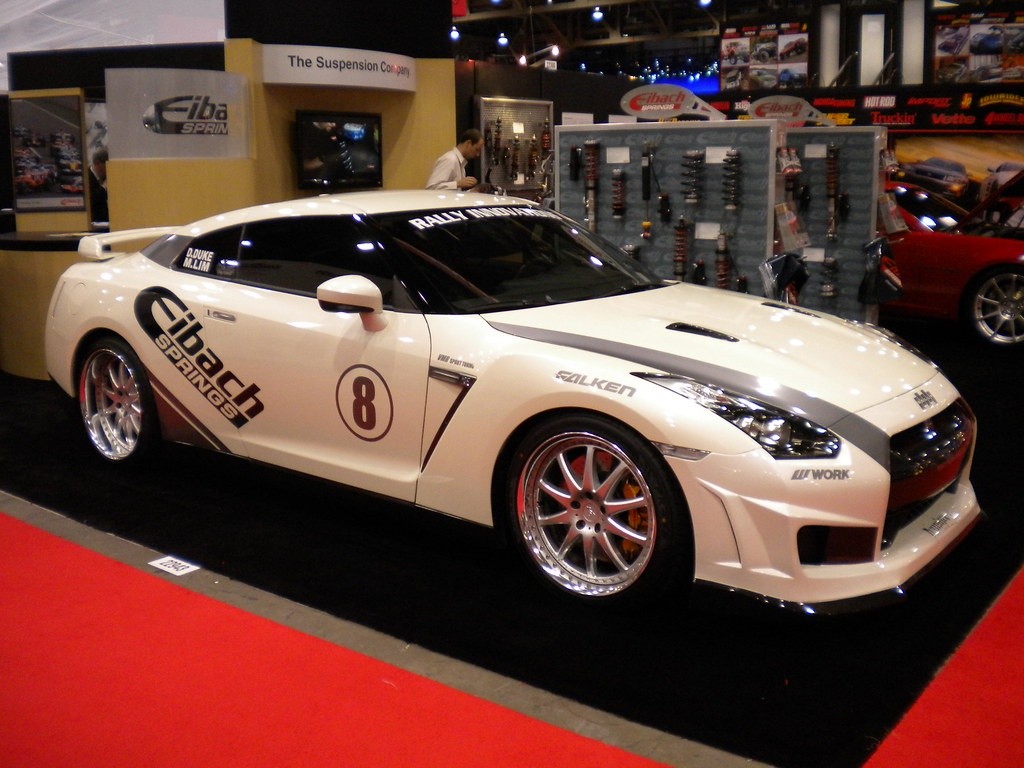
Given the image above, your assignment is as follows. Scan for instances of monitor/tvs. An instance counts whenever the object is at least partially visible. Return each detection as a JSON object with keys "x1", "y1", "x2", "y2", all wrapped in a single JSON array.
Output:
[{"x1": 289, "y1": 109, "x2": 384, "y2": 190}]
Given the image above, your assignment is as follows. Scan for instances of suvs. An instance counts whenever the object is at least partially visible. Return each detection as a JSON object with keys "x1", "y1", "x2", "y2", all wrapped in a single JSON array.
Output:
[{"x1": 726, "y1": 71, "x2": 741, "y2": 89}]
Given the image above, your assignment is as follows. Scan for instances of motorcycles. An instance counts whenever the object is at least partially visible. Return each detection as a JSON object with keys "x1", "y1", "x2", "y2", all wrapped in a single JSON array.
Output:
[
  {"x1": 723, "y1": 41, "x2": 750, "y2": 64},
  {"x1": 751, "y1": 42, "x2": 778, "y2": 63}
]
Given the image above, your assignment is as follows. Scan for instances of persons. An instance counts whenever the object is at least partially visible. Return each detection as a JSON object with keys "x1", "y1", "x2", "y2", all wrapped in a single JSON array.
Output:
[
  {"x1": 87, "y1": 147, "x2": 110, "y2": 233},
  {"x1": 425, "y1": 128, "x2": 485, "y2": 191},
  {"x1": 301, "y1": 122, "x2": 351, "y2": 172}
]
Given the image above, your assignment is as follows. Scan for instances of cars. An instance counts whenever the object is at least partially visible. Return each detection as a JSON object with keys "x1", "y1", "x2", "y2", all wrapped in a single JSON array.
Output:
[
  {"x1": 777, "y1": 69, "x2": 807, "y2": 85},
  {"x1": 877, "y1": 178, "x2": 1024, "y2": 345},
  {"x1": 750, "y1": 70, "x2": 778, "y2": 89},
  {"x1": 46, "y1": 190, "x2": 986, "y2": 614},
  {"x1": 891, "y1": 155, "x2": 973, "y2": 202},
  {"x1": 976, "y1": 64, "x2": 1003, "y2": 81}
]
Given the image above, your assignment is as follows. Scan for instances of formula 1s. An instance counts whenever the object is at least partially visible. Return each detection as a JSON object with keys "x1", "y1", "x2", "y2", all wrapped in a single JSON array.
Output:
[{"x1": 13, "y1": 124, "x2": 86, "y2": 194}]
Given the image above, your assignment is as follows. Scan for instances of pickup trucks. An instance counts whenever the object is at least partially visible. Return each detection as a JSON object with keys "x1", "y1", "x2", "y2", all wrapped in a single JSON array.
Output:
[{"x1": 781, "y1": 37, "x2": 807, "y2": 61}]
